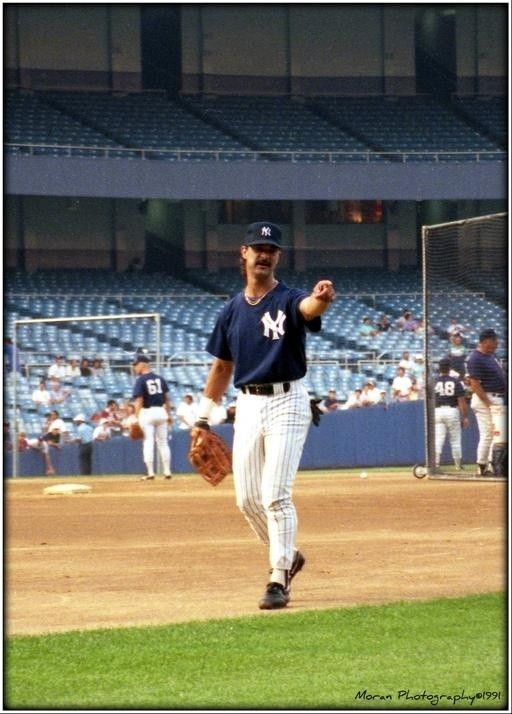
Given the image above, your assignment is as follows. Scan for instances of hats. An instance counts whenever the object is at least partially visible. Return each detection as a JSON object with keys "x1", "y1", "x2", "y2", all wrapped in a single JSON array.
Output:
[
  {"x1": 245, "y1": 222, "x2": 281, "y2": 248},
  {"x1": 329, "y1": 382, "x2": 387, "y2": 394},
  {"x1": 439, "y1": 360, "x2": 451, "y2": 368},
  {"x1": 480, "y1": 330, "x2": 496, "y2": 343},
  {"x1": 133, "y1": 355, "x2": 149, "y2": 365}
]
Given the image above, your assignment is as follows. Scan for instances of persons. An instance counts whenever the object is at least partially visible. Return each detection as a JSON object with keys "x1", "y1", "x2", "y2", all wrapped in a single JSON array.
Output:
[{"x1": 189, "y1": 221, "x2": 337, "y2": 609}]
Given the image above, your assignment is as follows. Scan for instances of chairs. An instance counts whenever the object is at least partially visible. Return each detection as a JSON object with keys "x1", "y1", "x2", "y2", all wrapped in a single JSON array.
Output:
[
  {"x1": 10, "y1": 263, "x2": 248, "y2": 444},
  {"x1": 276, "y1": 263, "x2": 509, "y2": 401}
]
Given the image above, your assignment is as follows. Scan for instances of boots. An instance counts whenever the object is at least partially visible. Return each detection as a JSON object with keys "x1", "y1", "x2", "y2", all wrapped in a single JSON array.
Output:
[{"x1": 429, "y1": 456, "x2": 507, "y2": 476}]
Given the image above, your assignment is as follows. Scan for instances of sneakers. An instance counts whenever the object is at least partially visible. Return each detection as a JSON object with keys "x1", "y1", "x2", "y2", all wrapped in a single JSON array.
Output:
[
  {"x1": 259, "y1": 550, "x2": 305, "y2": 609},
  {"x1": 140, "y1": 476, "x2": 172, "y2": 480}
]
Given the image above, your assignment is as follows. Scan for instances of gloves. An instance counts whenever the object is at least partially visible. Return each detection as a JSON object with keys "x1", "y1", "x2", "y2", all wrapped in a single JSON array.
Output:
[
  {"x1": 311, "y1": 399, "x2": 323, "y2": 426},
  {"x1": 195, "y1": 397, "x2": 217, "y2": 430}
]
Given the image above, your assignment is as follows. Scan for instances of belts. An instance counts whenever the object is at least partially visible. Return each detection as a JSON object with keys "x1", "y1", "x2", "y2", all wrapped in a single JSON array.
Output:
[
  {"x1": 493, "y1": 394, "x2": 504, "y2": 398},
  {"x1": 241, "y1": 383, "x2": 290, "y2": 395}
]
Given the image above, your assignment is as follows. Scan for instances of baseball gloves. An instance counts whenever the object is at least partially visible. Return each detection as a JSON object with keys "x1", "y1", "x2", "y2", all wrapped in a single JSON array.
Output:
[
  {"x1": 189, "y1": 431, "x2": 232, "y2": 486},
  {"x1": 132, "y1": 425, "x2": 144, "y2": 440}
]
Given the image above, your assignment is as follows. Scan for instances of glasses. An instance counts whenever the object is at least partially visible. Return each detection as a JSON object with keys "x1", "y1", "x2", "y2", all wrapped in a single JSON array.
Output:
[{"x1": 251, "y1": 246, "x2": 276, "y2": 254}]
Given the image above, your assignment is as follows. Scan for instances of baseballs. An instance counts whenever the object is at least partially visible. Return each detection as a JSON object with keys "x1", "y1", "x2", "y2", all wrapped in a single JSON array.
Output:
[{"x1": 360, "y1": 471, "x2": 368, "y2": 479}]
